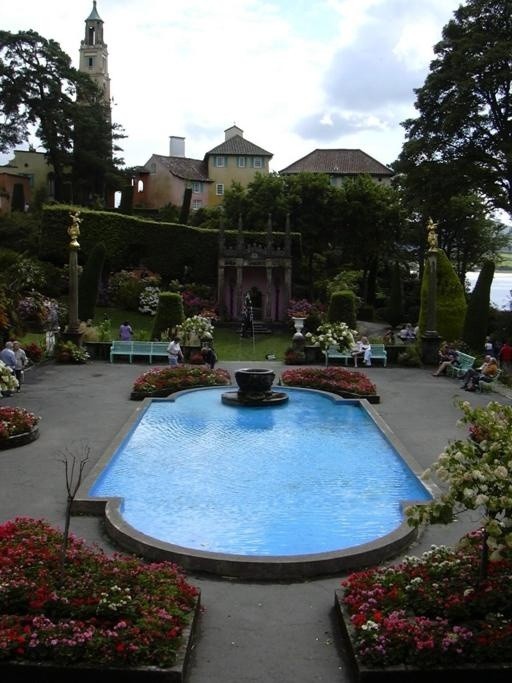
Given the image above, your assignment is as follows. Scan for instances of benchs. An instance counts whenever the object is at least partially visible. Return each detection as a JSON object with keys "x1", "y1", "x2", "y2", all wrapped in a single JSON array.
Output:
[
  {"x1": 479, "y1": 368, "x2": 501, "y2": 392},
  {"x1": 450, "y1": 350, "x2": 475, "y2": 379},
  {"x1": 111, "y1": 340, "x2": 172, "y2": 363},
  {"x1": 323, "y1": 344, "x2": 388, "y2": 366}
]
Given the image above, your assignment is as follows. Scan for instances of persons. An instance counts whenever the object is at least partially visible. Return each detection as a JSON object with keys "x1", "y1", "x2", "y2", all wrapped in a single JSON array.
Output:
[
  {"x1": 383, "y1": 321, "x2": 420, "y2": 344},
  {"x1": 356, "y1": 334, "x2": 373, "y2": 369},
  {"x1": 0, "y1": 340, "x2": 17, "y2": 397},
  {"x1": 493, "y1": 339, "x2": 502, "y2": 367},
  {"x1": 464, "y1": 355, "x2": 498, "y2": 391},
  {"x1": 484, "y1": 339, "x2": 494, "y2": 356},
  {"x1": 165, "y1": 334, "x2": 185, "y2": 366},
  {"x1": 456, "y1": 354, "x2": 492, "y2": 388},
  {"x1": 200, "y1": 346, "x2": 218, "y2": 370},
  {"x1": 118, "y1": 320, "x2": 134, "y2": 341},
  {"x1": 497, "y1": 339, "x2": 512, "y2": 375},
  {"x1": 11, "y1": 339, "x2": 28, "y2": 392},
  {"x1": 438, "y1": 344, "x2": 449, "y2": 375},
  {"x1": 432, "y1": 346, "x2": 461, "y2": 376}
]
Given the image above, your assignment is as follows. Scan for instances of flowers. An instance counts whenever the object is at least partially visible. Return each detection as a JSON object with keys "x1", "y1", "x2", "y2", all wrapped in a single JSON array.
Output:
[{"x1": 288, "y1": 298, "x2": 311, "y2": 317}]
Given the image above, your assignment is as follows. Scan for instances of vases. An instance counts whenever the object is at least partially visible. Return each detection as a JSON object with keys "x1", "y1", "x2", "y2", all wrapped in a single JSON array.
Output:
[
  {"x1": 235, "y1": 367, "x2": 275, "y2": 394},
  {"x1": 303, "y1": 344, "x2": 321, "y2": 363},
  {"x1": 292, "y1": 317, "x2": 306, "y2": 333}
]
